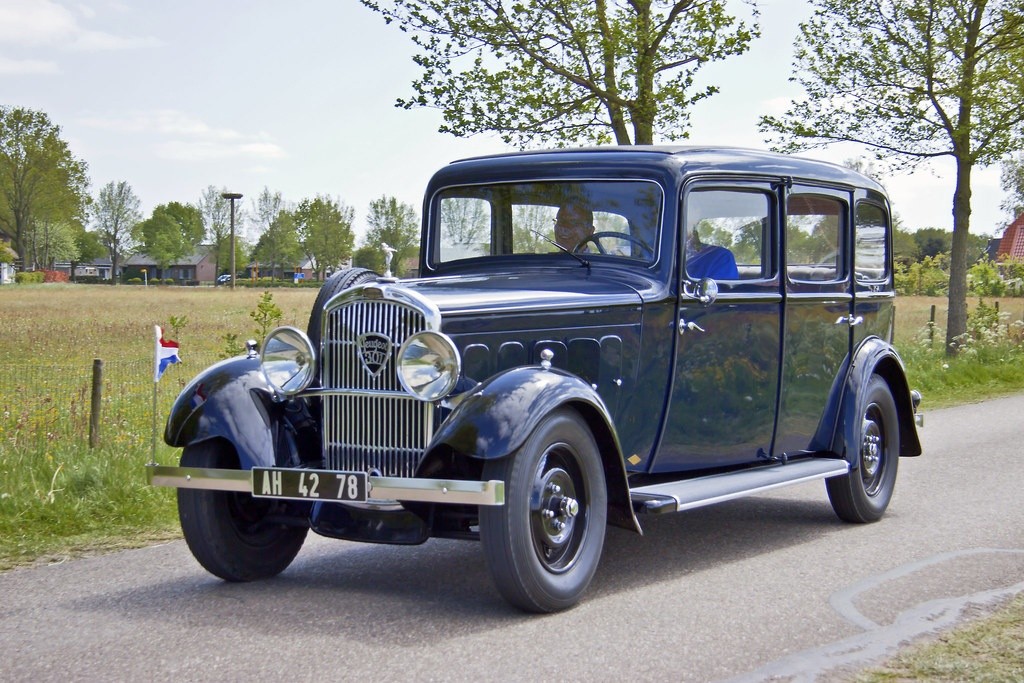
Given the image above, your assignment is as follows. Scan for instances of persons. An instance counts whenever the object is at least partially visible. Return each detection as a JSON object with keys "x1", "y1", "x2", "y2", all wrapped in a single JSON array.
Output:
[
  {"x1": 548, "y1": 200, "x2": 594, "y2": 253},
  {"x1": 643, "y1": 194, "x2": 738, "y2": 289}
]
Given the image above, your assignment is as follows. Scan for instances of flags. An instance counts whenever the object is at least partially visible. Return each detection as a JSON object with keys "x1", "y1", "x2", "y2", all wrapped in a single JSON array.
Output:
[{"x1": 153, "y1": 324, "x2": 182, "y2": 380}]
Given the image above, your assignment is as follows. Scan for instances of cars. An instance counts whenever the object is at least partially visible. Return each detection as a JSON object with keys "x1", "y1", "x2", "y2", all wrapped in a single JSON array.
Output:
[{"x1": 143, "y1": 143, "x2": 924, "y2": 608}]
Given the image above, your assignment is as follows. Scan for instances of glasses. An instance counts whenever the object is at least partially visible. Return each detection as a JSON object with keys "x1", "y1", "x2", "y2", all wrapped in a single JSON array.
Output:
[{"x1": 551, "y1": 218, "x2": 582, "y2": 225}]
